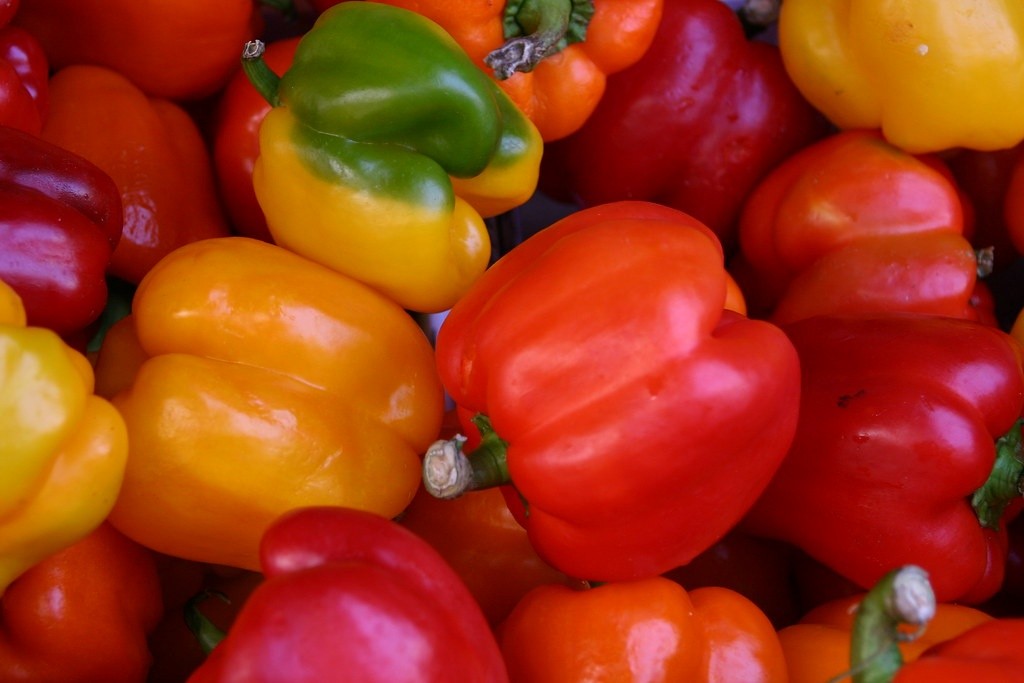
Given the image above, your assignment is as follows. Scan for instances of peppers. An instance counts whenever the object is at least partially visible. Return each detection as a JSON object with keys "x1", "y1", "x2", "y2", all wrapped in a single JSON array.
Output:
[{"x1": 0, "y1": 0, "x2": 1024, "y2": 683}]
